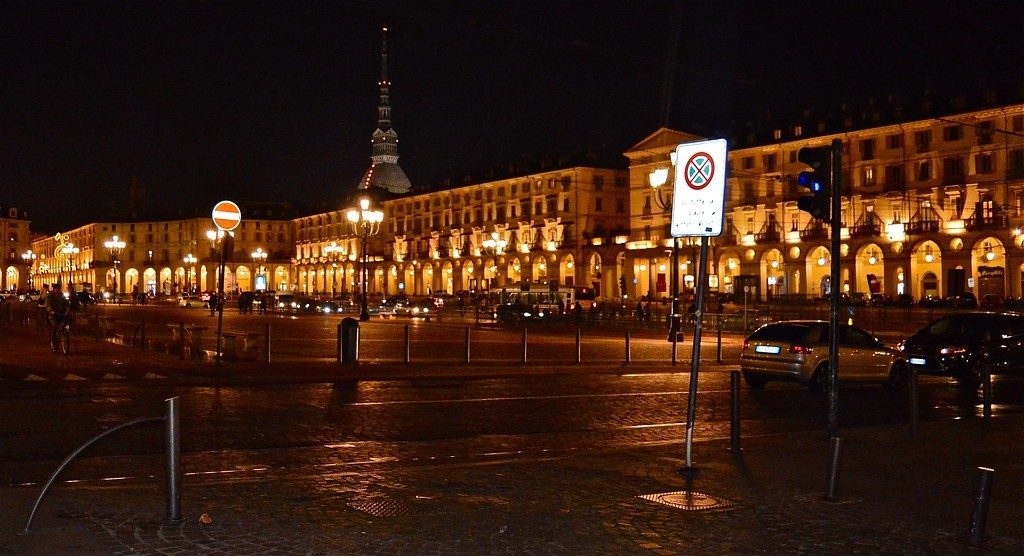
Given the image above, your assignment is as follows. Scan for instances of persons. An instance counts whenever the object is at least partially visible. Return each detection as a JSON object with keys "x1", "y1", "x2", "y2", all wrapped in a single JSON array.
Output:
[
  {"x1": 573, "y1": 301, "x2": 584, "y2": 318},
  {"x1": 22, "y1": 292, "x2": 33, "y2": 326},
  {"x1": 38, "y1": 284, "x2": 49, "y2": 329},
  {"x1": 82, "y1": 289, "x2": 89, "y2": 308},
  {"x1": 244, "y1": 292, "x2": 254, "y2": 314},
  {"x1": 259, "y1": 292, "x2": 267, "y2": 315},
  {"x1": 47, "y1": 284, "x2": 68, "y2": 355},
  {"x1": 68, "y1": 286, "x2": 79, "y2": 324},
  {"x1": 238, "y1": 294, "x2": 245, "y2": 315},
  {"x1": 644, "y1": 303, "x2": 652, "y2": 320},
  {"x1": 586, "y1": 303, "x2": 596, "y2": 325},
  {"x1": 687, "y1": 300, "x2": 696, "y2": 326},
  {"x1": 647, "y1": 290, "x2": 652, "y2": 303},
  {"x1": 637, "y1": 301, "x2": 644, "y2": 328},
  {"x1": 715, "y1": 298, "x2": 726, "y2": 326},
  {"x1": 558, "y1": 298, "x2": 565, "y2": 313},
  {"x1": 209, "y1": 291, "x2": 217, "y2": 317}
]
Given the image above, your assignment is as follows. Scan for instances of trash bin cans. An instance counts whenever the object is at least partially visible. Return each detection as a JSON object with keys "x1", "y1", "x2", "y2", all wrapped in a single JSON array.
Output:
[
  {"x1": 118, "y1": 297, "x2": 122, "y2": 304},
  {"x1": 337, "y1": 318, "x2": 360, "y2": 362}
]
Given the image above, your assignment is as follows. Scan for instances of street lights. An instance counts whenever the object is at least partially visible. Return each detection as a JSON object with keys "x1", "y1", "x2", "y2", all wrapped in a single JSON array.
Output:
[
  {"x1": 62, "y1": 243, "x2": 79, "y2": 290},
  {"x1": 482, "y1": 231, "x2": 508, "y2": 289},
  {"x1": 324, "y1": 240, "x2": 344, "y2": 300},
  {"x1": 105, "y1": 235, "x2": 127, "y2": 304},
  {"x1": 250, "y1": 247, "x2": 268, "y2": 296},
  {"x1": 20, "y1": 250, "x2": 37, "y2": 290},
  {"x1": 650, "y1": 148, "x2": 685, "y2": 342},
  {"x1": 182, "y1": 253, "x2": 197, "y2": 297},
  {"x1": 346, "y1": 196, "x2": 384, "y2": 321}
]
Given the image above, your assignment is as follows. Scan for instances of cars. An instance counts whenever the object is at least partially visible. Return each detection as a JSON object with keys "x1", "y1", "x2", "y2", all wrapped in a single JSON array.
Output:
[
  {"x1": 0, "y1": 288, "x2": 16, "y2": 304},
  {"x1": 492, "y1": 302, "x2": 544, "y2": 323},
  {"x1": 68, "y1": 291, "x2": 99, "y2": 306},
  {"x1": 899, "y1": 311, "x2": 1024, "y2": 390},
  {"x1": 19, "y1": 289, "x2": 42, "y2": 304},
  {"x1": 176, "y1": 289, "x2": 478, "y2": 316},
  {"x1": 739, "y1": 318, "x2": 911, "y2": 402},
  {"x1": 826, "y1": 289, "x2": 1024, "y2": 313}
]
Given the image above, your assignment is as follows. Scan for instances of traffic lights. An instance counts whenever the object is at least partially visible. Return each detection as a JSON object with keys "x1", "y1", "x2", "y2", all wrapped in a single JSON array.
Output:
[
  {"x1": 796, "y1": 145, "x2": 831, "y2": 224},
  {"x1": 619, "y1": 275, "x2": 625, "y2": 289}
]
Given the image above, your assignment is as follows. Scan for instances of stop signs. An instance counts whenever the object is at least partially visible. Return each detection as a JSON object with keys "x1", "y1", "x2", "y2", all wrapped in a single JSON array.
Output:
[
  {"x1": 869, "y1": 275, "x2": 877, "y2": 289},
  {"x1": 212, "y1": 200, "x2": 242, "y2": 231}
]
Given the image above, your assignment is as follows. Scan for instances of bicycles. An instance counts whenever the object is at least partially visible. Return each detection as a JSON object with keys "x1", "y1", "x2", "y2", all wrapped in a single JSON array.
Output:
[{"x1": 43, "y1": 308, "x2": 75, "y2": 357}]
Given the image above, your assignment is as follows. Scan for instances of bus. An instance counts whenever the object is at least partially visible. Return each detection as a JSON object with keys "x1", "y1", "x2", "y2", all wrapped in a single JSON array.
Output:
[{"x1": 478, "y1": 284, "x2": 595, "y2": 315}]
